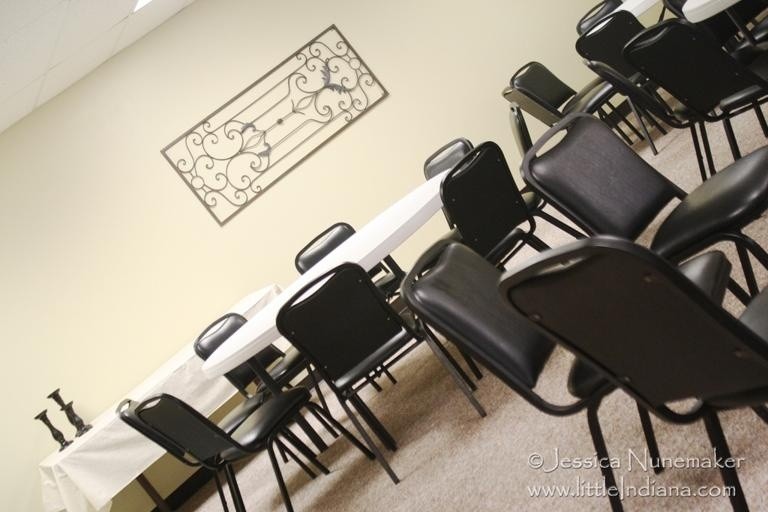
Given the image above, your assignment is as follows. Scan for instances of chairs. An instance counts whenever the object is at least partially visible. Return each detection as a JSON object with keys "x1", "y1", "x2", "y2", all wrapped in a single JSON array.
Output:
[{"x1": 115, "y1": 0, "x2": 768, "y2": 511}]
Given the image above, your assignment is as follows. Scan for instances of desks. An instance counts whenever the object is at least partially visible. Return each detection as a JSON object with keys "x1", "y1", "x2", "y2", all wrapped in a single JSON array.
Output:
[{"x1": 37, "y1": 284, "x2": 277, "y2": 510}]
